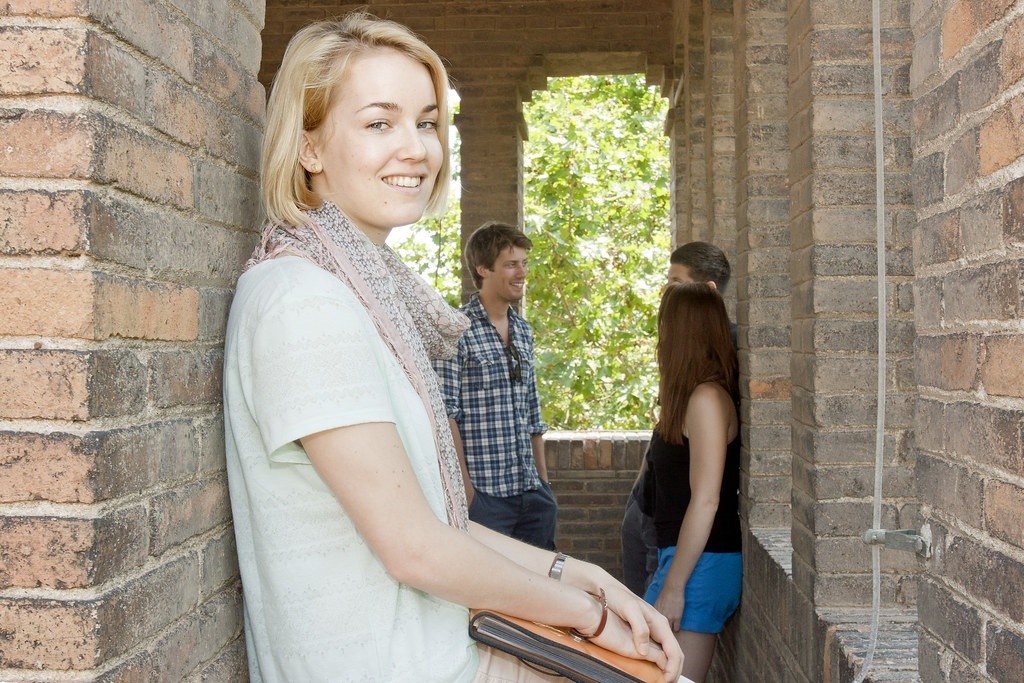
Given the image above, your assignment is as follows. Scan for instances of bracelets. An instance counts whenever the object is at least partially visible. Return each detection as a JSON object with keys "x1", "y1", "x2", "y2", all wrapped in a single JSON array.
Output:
[
  {"x1": 548, "y1": 551, "x2": 570, "y2": 581},
  {"x1": 570, "y1": 588, "x2": 609, "y2": 639}
]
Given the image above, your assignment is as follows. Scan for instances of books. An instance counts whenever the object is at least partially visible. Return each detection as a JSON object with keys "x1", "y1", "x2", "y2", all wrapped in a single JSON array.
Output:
[{"x1": 468, "y1": 608, "x2": 667, "y2": 683}]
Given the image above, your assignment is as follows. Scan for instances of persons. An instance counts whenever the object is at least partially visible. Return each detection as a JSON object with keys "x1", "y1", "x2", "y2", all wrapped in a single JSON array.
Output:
[
  {"x1": 433, "y1": 220, "x2": 559, "y2": 553},
  {"x1": 222, "y1": 6, "x2": 685, "y2": 683},
  {"x1": 619, "y1": 240, "x2": 743, "y2": 683}
]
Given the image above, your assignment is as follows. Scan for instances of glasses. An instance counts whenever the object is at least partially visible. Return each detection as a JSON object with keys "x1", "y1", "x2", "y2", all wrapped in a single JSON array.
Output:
[{"x1": 511, "y1": 342, "x2": 525, "y2": 382}]
